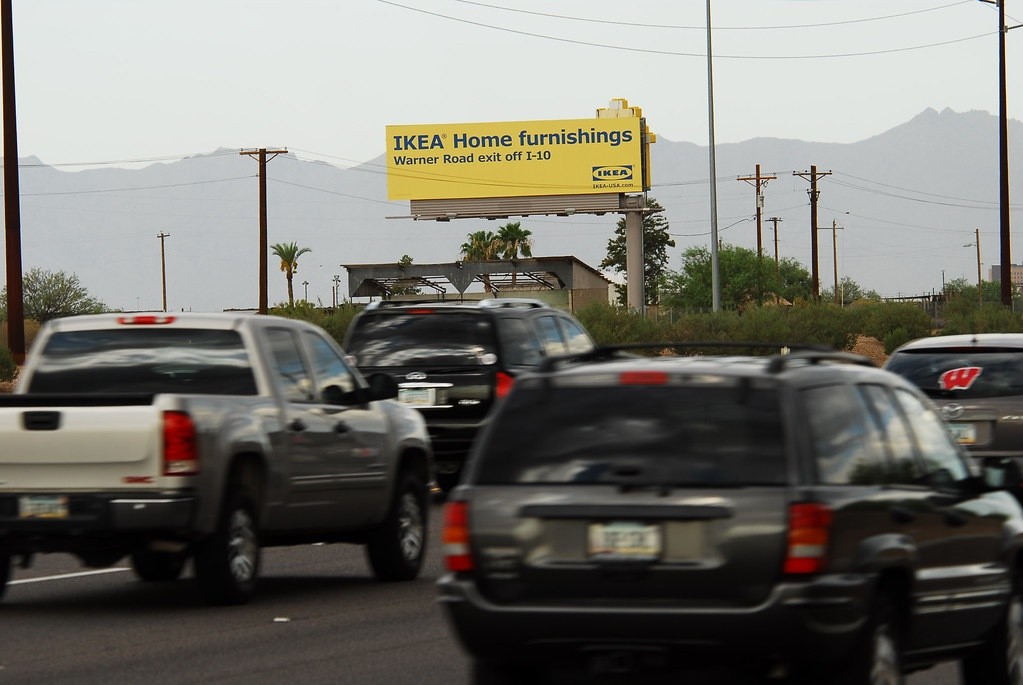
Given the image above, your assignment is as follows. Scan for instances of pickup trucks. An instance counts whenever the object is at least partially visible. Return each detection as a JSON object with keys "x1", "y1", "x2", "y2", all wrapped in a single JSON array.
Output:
[{"x1": 1, "y1": 316, "x2": 437, "y2": 601}]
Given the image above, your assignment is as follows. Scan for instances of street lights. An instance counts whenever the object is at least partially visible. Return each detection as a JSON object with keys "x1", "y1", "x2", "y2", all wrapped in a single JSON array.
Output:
[
  {"x1": 333, "y1": 274, "x2": 342, "y2": 308},
  {"x1": 302, "y1": 281, "x2": 310, "y2": 304},
  {"x1": 964, "y1": 242, "x2": 983, "y2": 305},
  {"x1": 157, "y1": 230, "x2": 175, "y2": 312}
]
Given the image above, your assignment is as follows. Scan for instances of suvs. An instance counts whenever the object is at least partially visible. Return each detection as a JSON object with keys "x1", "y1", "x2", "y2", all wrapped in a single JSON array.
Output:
[
  {"x1": 439, "y1": 340, "x2": 1022, "y2": 683},
  {"x1": 340, "y1": 297, "x2": 598, "y2": 502}
]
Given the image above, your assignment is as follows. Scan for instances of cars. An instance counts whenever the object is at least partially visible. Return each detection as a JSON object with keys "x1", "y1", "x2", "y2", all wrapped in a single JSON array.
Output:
[{"x1": 870, "y1": 335, "x2": 1022, "y2": 480}]
